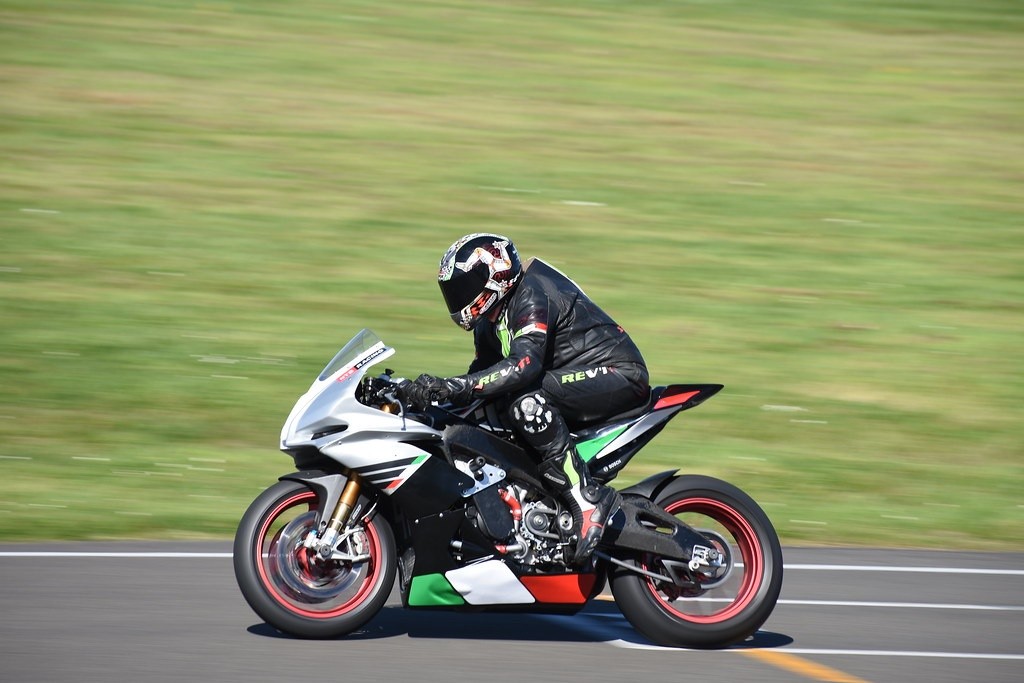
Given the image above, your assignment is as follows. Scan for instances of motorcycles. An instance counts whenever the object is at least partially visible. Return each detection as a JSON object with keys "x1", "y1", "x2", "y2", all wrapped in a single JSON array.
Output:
[{"x1": 233, "y1": 328, "x2": 785, "y2": 651}]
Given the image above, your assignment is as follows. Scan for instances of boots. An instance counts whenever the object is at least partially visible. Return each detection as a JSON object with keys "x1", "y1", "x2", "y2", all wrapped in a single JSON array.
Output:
[{"x1": 537, "y1": 439, "x2": 624, "y2": 565}]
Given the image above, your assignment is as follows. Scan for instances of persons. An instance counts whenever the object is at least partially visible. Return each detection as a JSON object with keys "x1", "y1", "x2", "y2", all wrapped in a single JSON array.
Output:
[{"x1": 405, "y1": 232, "x2": 650, "y2": 568}]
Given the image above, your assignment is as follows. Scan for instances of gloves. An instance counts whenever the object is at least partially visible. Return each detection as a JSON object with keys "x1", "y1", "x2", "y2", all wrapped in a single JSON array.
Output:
[{"x1": 405, "y1": 373, "x2": 466, "y2": 409}]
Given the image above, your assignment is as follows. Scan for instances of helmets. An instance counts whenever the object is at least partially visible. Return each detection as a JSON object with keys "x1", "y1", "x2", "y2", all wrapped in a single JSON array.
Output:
[{"x1": 438, "y1": 232, "x2": 523, "y2": 331}]
